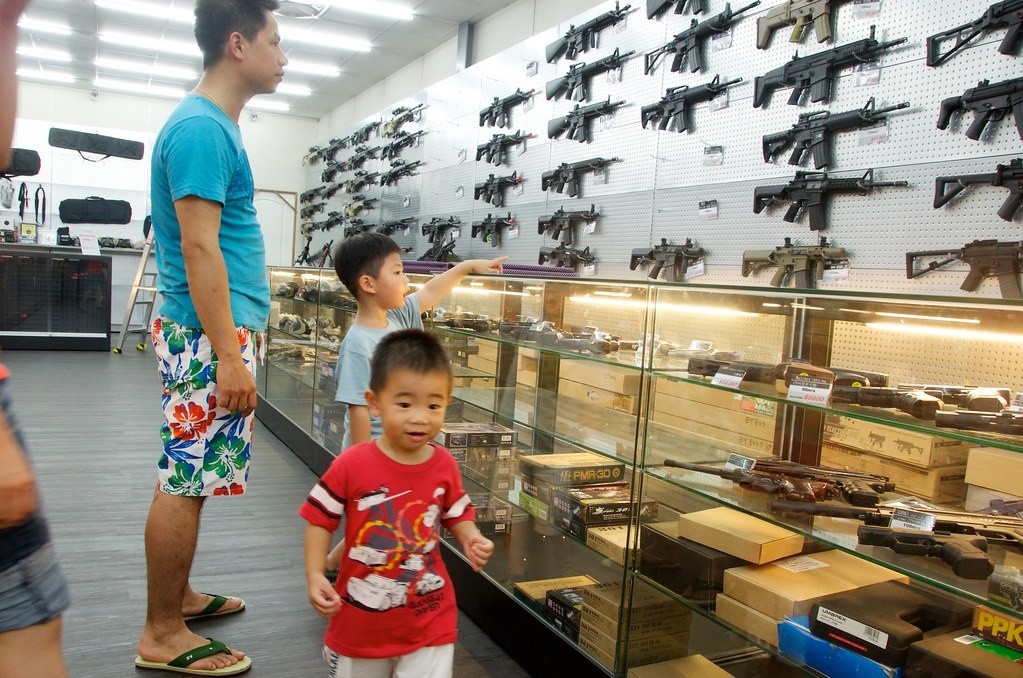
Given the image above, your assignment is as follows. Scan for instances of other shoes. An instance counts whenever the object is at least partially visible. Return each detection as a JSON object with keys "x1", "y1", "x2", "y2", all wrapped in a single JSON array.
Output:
[{"x1": 322, "y1": 563, "x2": 341, "y2": 582}]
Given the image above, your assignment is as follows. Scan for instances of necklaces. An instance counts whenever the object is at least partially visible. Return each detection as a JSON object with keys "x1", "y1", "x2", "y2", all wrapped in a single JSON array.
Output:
[{"x1": 195, "y1": 86, "x2": 223, "y2": 111}]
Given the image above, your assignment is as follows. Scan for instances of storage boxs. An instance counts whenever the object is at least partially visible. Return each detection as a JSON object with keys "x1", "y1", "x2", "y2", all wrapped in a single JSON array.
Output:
[
  {"x1": 433, "y1": 329, "x2": 1023, "y2": 678},
  {"x1": 312, "y1": 354, "x2": 346, "y2": 457}
]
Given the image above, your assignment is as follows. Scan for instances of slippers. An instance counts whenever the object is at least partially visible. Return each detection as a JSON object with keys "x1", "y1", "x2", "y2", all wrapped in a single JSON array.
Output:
[
  {"x1": 182, "y1": 592, "x2": 246, "y2": 622},
  {"x1": 134, "y1": 637, "x2": 252, "y2": 676}
]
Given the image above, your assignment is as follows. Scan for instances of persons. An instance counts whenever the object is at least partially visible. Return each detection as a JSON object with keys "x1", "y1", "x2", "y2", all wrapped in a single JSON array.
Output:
[
  {"x1": 324, "y1": 233, "x2": 509, "y2": 581},
  {"x1": 131, "y1": 0, "x2": 287, "y2": 676},
  {"x1": 0, "y1": 0, "x2": 73, "y2": 678},
  {"x1": 297, "y1": 328, "x2": 495, "y2": 678}
]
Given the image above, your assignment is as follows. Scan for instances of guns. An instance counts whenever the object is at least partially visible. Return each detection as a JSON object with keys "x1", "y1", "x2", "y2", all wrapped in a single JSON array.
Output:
[
  {"x1": 422, "y1": 308, "x2": 1023, "y2": 611},
  {"x1": 298, "y1": 0, "x2": 1023, "y2": 301}
]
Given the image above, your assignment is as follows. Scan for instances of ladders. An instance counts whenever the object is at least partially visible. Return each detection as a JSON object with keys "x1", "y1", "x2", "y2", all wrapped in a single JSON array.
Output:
[{"x1": 112, "y1": 225, "x2": 159, "y2": 355}]
